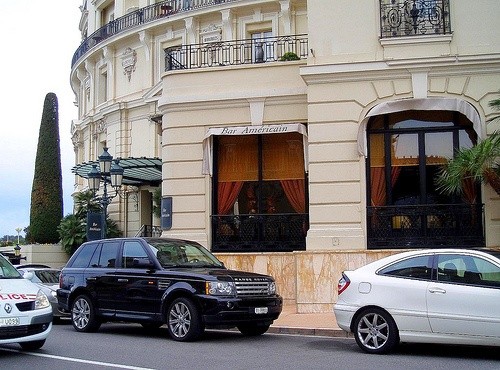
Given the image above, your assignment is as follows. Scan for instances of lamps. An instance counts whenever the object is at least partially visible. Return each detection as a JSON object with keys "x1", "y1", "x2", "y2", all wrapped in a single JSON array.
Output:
[{"x1": 14, "y1": 243, "x2": 26, "y2": 260}]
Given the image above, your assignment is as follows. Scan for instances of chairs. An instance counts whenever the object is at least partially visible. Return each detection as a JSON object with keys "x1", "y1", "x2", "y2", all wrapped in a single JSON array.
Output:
[
  {"x1": 157, "y1": 251, "x2": 171, "y2": 265},
  {"x1": 464, "y1": 264, "x2": 482, "y2": 285},
  {"x1": 442, "y1": 263, "x2": 464, "y2": 282}
]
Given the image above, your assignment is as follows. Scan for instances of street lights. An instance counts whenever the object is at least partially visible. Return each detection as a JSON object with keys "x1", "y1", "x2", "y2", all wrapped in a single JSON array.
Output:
[{"x1": 88, "y1": 147, "x2": 124, "y2": 239}]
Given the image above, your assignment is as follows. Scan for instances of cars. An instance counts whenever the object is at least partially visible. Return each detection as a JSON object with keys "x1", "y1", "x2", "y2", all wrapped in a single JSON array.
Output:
[
  {"x1": 333, "y1": 248, "x2": 500, "y2": 355},
  {"x1": 0, "y1": 253, "x2": 71, "y2": 351}
]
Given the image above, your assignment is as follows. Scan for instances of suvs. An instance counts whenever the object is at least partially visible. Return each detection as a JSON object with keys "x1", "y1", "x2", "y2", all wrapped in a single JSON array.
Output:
[{"x1": 56, "y1": 237, "x2": 283, "y2": 342}]
